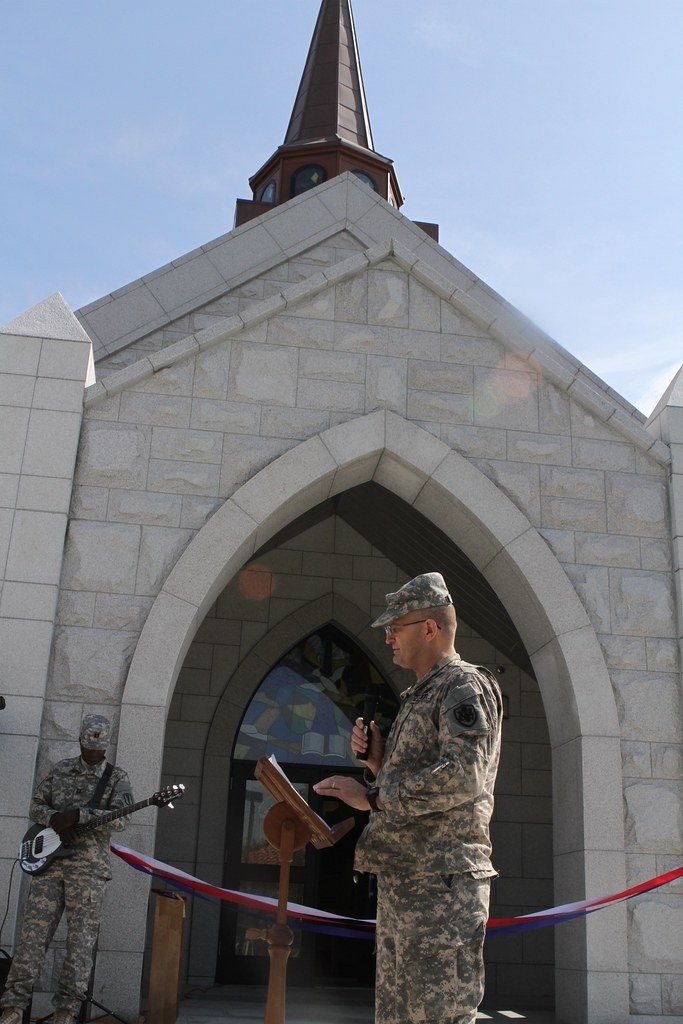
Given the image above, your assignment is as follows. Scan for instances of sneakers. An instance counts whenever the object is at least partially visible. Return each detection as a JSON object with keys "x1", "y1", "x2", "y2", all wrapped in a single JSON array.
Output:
[
  {"x1": 0, "y1": 1007, "x2": 23, "y2": 1024},
  {"x1": 51, "y1": 1015, "x2": 76, "y2": 1024}
]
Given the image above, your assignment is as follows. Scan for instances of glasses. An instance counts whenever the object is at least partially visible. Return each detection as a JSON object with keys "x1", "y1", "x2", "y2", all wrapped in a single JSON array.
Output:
[{"x1": 384, "y1": 620, "x2": 443, "y2": 638}]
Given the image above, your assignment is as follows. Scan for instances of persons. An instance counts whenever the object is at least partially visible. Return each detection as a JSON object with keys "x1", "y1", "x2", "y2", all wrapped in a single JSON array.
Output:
[
  {"x1": 311, "y1": 570, "x2": 508, "y2": 1024},
  {"x1": 0, "y1": 710, "x2": 137, "y2": 1024}
]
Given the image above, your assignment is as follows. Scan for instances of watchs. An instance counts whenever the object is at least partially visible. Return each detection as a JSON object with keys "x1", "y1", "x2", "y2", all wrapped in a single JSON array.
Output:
[{"x1": 366, "y1": 786, "x2": 384, "y2": 813}]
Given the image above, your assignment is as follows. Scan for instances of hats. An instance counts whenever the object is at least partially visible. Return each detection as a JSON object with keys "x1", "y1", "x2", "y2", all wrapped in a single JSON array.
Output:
[
  {"x1": 370, "y1": 572, "x2": 453, "y2": 627},
  {"x1": 78, "y1": 714, "x2": 112, "y2": 751}
]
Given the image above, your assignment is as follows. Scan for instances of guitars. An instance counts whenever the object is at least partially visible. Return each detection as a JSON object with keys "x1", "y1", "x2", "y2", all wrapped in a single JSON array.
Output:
[{"x1": 18, "y1": 782, "x2": 186, "y2": 876}]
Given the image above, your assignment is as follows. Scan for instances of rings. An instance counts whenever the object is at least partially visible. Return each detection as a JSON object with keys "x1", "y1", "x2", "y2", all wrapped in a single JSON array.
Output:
[{"x1": 331, "y1": 780, "x2": 337, "y2": 789}]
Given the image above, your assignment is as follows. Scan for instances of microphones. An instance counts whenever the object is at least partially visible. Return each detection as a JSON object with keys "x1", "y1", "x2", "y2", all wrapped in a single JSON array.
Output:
[{"x1": 355, "y1": 682, "x2": 380, "y2": 760}]
